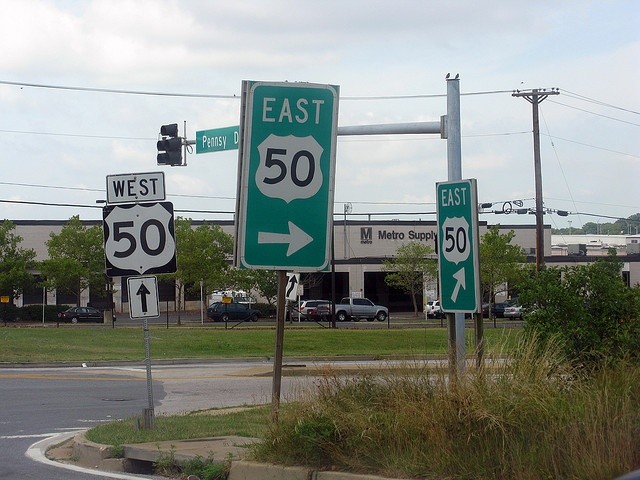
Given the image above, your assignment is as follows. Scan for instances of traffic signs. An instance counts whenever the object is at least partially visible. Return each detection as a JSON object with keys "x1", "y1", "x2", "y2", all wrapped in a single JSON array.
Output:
[
  {"x1": 102, "y1": 201, "x2": 179, "y2": 277},
  {"x1": 241, "y1": 80, "x2": 338, "y2": 273},
  {"x1": 437, "y1": 178, "x2": 481, "y2": 315}
]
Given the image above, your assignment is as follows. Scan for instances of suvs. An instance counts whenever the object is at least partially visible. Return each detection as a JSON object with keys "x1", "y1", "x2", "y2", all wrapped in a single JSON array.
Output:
[
  {"x1": 424, "y1": 300, "x2": 441, "y2": 318},
  {"x1": 207, "y1": 302, "x2": 263, "y2": 322}
]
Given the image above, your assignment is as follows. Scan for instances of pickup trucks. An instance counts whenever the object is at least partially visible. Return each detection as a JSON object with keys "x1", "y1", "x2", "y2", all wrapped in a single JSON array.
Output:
[{"x1": 318, "y1": 297, "x2": 388, "y2": 322}]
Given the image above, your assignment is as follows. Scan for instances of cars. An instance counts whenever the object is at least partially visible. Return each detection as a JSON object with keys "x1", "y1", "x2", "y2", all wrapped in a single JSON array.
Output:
[
  {"x1": 503, "y1": 304, "x2": 537, "y2": 320},
  {"x1": 504, "y1": 298, "x2": 521, "y2": 304},
  {"x1": 481, "y1": 304, "x2": 508, "y2": 318},
  {"x1": 292, "y1": 299, "x2": 334, "y2": 321},
  {"x1": 58, "y1": 307, "x2": 116, "y2": 324}
]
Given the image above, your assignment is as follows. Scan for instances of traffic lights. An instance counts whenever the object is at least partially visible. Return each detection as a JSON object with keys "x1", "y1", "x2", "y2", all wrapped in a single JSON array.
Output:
[{"x1": 155, "y1": 123, "x2": 183, "y2": 167}]
